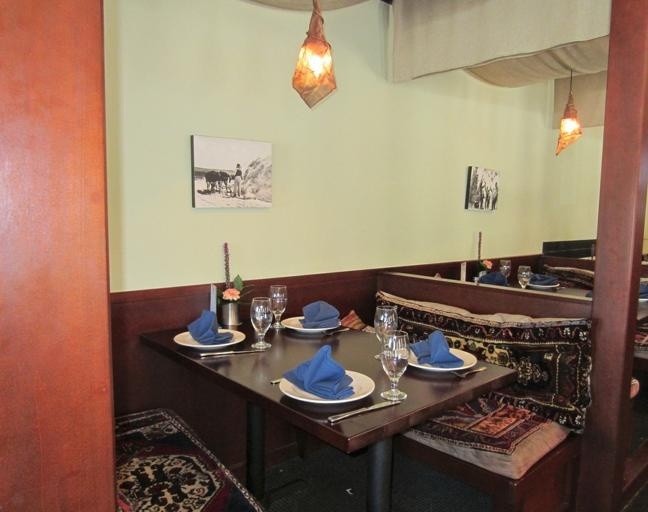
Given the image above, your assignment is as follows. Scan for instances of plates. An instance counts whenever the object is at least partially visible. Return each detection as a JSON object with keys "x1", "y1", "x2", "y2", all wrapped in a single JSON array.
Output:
[
  {"x1": 395, "y1": 347, "x2": 478, "y2": 373},
  {"x1": 281, "y1": 316, "x2": 342, "y2": 334},
  {"x1": 526, "y1": 278, "x2": 561, "y2": 289},
  {"x1": 278, "y1": 369, "x2": 376, "y2": 405},
  {"x1": 173, "y1": 328, "x2": 246, "y2": 349}
]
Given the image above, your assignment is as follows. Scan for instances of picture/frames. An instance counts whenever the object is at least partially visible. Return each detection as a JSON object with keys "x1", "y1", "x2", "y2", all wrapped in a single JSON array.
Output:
[
  {"x1": 465, "y1": 164, "x2": 498, "y2": 211},
  {"x1": 191, "y1": 134, "x2": 273, "y2": 208}
]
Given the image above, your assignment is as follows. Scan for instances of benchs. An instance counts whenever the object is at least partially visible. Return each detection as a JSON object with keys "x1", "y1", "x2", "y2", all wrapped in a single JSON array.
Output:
[
  {"x1": 540, "y1": 263, "x2": 648, "y2": 358},
  {"x1": 114, "y1": 406, "x2": 271, "y2": 512},
  {"x1": 378, "y1": 271, "x2": 591, "y2": 512}
]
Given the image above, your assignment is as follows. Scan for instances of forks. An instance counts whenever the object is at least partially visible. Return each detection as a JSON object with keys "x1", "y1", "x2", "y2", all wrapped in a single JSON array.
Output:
[
  {"x1": 321, "y1": 328, "x2": 350, "y2": 336},
  {"x1": 449, "y1": 365, "x2": 489, "y2": 378}
]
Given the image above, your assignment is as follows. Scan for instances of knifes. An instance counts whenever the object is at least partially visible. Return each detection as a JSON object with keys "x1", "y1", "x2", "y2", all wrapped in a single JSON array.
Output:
[
  {"x1": 201, "y1": 348, "x2": 266, "y2": 357},
  {"x1": 327, "y1": 400, "x2": 403, "y2": 423}
]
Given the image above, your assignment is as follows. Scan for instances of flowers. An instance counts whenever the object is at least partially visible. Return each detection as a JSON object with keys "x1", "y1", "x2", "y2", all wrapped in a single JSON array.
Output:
[
  {"x1": 215, "y1": 275, "x2": 243, "y2": 303},
  {"x1": 477, "y1": 260, "x2": 494, "y2": 272}
]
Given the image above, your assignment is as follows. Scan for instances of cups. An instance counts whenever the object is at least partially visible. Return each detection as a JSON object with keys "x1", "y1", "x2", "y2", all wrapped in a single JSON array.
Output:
[
  {"x1": 517, "y1": 265, "x2": 531, "y2": 289},
  {"x1": 380, "y1": 329, "x2": 410, "y2": 400},
  {"x1": 250, "y1": 296, "x2": 272, "y2": 349},
  {"x1": 499, "y1": 259, "x2": 512, "y2": 287},
  {"x1": 270, "y1": 284, "x2": 288, "y2": 327},
  {"x1": 374, "y1": 305, "x2": 399, "y2": 360}
]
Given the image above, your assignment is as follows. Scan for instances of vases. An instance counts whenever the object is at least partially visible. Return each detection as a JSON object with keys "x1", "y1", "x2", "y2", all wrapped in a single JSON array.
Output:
[{"x1": 223, "y1": 302, "x2": 239, "y2": 331}]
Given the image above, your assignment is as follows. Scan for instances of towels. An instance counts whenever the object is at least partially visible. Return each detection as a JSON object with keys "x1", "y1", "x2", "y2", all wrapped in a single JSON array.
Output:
[
  {"x1": 529, "y1": 274, "x2": 558, "y2": 285},
  {"x1": 282, "y1": 345, "x2": 355, "y2": 400},
  {"x1": 409, "y1": 330, "x2": 464, "y2": 368},
  {"x1": 188, "y1": 309, "x2": 234, "y2": 345},
  {"x1": 638, "y1": 281, "x2": 647, "y2": 299},
  {"x1": 478, "y1": 271, "x2": 507, "y2": 285},
  {"x1": 299, "y1": 300, "x2": 340, "y2": 329}
]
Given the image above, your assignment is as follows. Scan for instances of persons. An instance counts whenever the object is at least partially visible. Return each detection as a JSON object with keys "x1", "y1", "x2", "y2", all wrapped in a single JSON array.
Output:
[
  {"x1": 233, "y1": 163, "x2": 243, "y2": 197},
  {"x1": 491, "y1": 182, "x2": 499, "y2": 208},
  {"x1": 478, "y1": 174, "x2": 485, "y2": 209}
]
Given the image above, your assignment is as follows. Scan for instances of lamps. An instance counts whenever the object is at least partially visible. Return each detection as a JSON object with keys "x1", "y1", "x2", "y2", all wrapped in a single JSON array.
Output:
[
  {"x1": 292, "y1": 0, "x2": 336, "y2": 106},
  {"x1": 553, "y1": 68, "x2": 581, "y2": 154}
]
